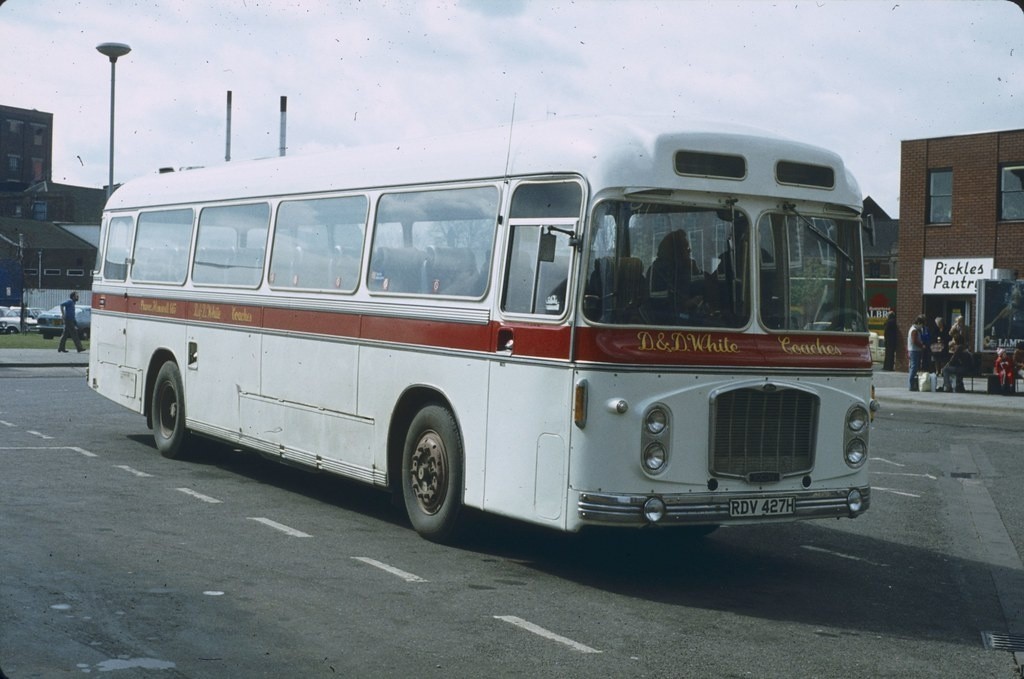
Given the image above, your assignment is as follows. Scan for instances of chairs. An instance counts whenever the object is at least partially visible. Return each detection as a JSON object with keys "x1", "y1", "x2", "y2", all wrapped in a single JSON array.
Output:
[
  {"x1": 419, "y1": 245, "x2": 479, "y2": 297},
  {"x1": 476, "y1": 249, "x2": 534, "y2": 315},
  {"x1": 128, "y1": 243, "x2": 189, "y2": 283},
  {"x1": 370, "y1": 247, "x2": 420, "y2": 294},
  {"x1": 288, "y1": 246, "x2": 327, "y2": 290},
  {"x1": 589, "y1": 256, "x2": 645, "y2": 325},
  {"x1": 224, "y1": 247, "x2": 259, "y2": 285},
  {"x1": 327, "y1": 245, "x2": 361, "y2": 290},
  {"x1": 540, "y1": 257, "x2": 570, "y2": 315},
  {"x1": 192, "y1": 248, "x2": 224, "y2": 284},
  {"x1": 104, "y1": 247, "x2": 127, "y2": 280}
]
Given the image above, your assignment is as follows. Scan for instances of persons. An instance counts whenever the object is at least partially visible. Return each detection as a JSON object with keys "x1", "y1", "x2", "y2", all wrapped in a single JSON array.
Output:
[
  {"x1": 58, "y1": 291, "x2": 86, "y2": 353},
  {"x1": 635, "y1": 227, "x2": 712, "y2": 327},
  {"x1": 1012, "y1": 341, "x2": 1024, "y2": 378},
  {"x1": 907, "y1": 314, "x2": 978, "y2": 392},
  {"x1": 687, "y1": 216, "x2": 786, "y2": 327},
  {"x1": 995, "y1": 347, "x2": 1014, "y2": 392},
  {"x1": 883, "y1": 310, "x2": 897, "y2": 370}
]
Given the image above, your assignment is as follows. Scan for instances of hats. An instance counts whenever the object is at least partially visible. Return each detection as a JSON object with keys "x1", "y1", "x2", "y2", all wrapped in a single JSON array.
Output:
[{"x1": 996, "y1": 348, "x2": 1005, "y2": 356}]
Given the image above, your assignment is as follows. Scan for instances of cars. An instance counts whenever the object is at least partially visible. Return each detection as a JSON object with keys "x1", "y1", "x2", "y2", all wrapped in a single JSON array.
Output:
[
  {"x1": 0, "y1": 306, "x2": 46, "y2": 335},
  {"x1": 35, "y1": 305, "x2": 91, "y2": 339}
]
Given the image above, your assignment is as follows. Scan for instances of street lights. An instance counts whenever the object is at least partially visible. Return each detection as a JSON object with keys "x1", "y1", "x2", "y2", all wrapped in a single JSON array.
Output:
[{"x1": 96, "y1": 43, "x2": 131, "y2": 198}]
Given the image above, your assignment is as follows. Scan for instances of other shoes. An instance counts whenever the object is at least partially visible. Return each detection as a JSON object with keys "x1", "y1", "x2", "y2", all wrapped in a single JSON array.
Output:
[
  {"x1": 78, "y1": 349, "x2": 86, "y2": 352},
  {"x1": 58, "y1": 349, "x2": 68, "y2": 352}
]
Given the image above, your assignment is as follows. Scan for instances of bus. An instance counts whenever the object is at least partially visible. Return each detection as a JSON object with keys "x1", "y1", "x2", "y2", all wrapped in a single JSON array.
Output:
[
  {"x1": 83, "y1": 120, "x2": 874, "y2": 543},
  {"x1": 789, "y1": 278, "x2": 896, "y2": 336}
]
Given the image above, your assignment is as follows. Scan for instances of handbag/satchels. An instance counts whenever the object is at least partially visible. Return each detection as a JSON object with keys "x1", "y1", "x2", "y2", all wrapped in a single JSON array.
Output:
[
  {"x1": 930, "y1": 373, "x2": 937, "y2": 391},
  {"x1": 918, "y1": 372, "x2": 930, "y2": 391}
]
